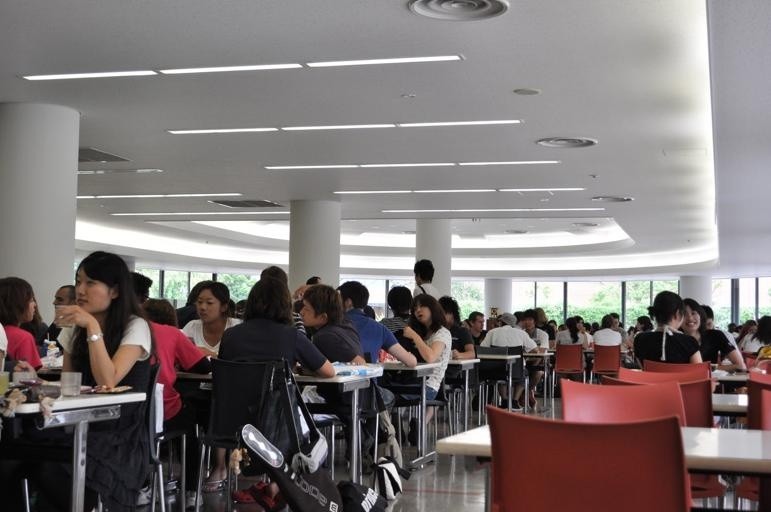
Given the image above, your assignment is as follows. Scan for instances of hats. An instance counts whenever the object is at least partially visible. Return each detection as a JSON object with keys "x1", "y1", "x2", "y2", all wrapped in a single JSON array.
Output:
[{"x1": 498, "y1": 312, "x2": 517, "y2": 328}]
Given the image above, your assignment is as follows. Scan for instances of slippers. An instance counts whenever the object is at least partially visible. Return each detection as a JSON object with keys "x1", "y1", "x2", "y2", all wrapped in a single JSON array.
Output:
[
  {"x1": 203, "y1": 472, "x2": 229, "y2": 492},
  {"x1": 233, "y1": 480, "x2": 287, "y2": 512}
]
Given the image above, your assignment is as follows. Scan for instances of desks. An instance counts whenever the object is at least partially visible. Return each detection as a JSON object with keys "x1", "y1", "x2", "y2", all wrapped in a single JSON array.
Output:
[{"x1": 15, "y1": 380, "x2": 147, "y2": 510}]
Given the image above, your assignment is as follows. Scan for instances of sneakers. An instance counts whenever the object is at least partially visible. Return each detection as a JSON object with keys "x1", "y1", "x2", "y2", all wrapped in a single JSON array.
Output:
[{"x1": 501, "y1": 398, "x2": 523, "y2": 412}]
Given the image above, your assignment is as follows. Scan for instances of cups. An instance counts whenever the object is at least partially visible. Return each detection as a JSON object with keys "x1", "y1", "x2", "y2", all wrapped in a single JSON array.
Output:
[
  {"x1": 0, "y1": 371, "x2": 10, "y2": 397},
  {"x1": 54, "y1": 303, "x2": 75, "y2": 329},
  {"x1": 12, "y1": 371, "x2": 37, "y2": 388},
  {"x1": 60, "y1": 371, "x2": 83, "y2": 397},
  {"x1": 41, "y1": 338, "x2": 61, "y2": 368}
]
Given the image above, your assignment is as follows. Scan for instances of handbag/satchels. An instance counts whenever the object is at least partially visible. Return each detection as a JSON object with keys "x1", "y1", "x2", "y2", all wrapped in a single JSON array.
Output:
[
  {"x1": 371, "y1": 409, "x2": 403, "y2": 471},
  {"x1": 292, "y1": 433, "x2": 330, "y2": 475},
  {"x1": 338, "y1": 480, "x2": 390, "y2": 511},
  {"x1": 372, "y1": 456, "x2": 403, "y2": 500}
]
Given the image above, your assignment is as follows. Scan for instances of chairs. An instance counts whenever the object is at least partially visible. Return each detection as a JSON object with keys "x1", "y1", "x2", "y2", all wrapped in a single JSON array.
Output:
[
  {"x1": 4, "y1": 420, "x2": 31, "y2": 512},
  {"x1": 95, "y1": 359, "x2": 480, "y2": 511},
  {"x1": 478, "y1": 341, "x2": 771, "y2": 512}
]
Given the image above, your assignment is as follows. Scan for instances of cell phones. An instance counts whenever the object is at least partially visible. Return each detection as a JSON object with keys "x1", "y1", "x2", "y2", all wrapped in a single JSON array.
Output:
[{"x1": 19, "y1": 379, "x2": 42, "y2": 386}]
